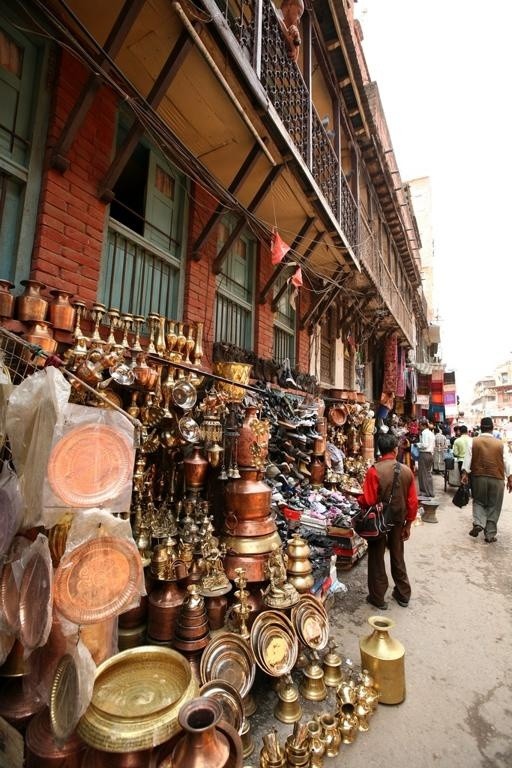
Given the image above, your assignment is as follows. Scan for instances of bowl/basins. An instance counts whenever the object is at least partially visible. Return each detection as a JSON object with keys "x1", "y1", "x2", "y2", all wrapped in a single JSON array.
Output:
[
  {"x1": 171, "y1": 379, "x2": 200, "y2": 442},
  {"x1": 109, "y1": 363, "x2": 135, "y2": 386}
]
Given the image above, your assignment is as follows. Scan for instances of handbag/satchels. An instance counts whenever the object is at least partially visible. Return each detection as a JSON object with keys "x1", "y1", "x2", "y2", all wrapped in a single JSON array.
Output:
[
  {"x1": 351, "y1": 497, "x2": 395, "y2": 541},
  {"x1": 452, "y1": 484, "x2": 471, "y2": 508}
]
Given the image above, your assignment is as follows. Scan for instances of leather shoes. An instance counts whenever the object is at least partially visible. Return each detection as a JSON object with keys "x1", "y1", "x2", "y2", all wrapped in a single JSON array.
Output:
[
  {"x1": 469, "y1": 525, "x2": 483, "y2": 538},
  {"x1": 484, "y1": 537, "x2": 498, "y2": 543}
]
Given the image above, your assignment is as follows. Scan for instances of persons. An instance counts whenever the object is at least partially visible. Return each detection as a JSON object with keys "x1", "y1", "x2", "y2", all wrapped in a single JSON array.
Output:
[
  {"x1": 358, "y1": 434, "x2": 418, "y2": 610},
  {"x1": 389, "y1": 415, "x2": 512, "y2": 496},
  {"x1": 467, "y1": 417, "x2": 512, "y2": 543}
]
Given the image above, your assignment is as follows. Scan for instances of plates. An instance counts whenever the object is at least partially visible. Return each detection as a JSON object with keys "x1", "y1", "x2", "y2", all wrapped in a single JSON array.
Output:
[
  {"x1": 0, "y1": 399, "x2": 143, "y2": 741},
  {"x1": 200, "y1": 593, "x2": 329, "y2": 736}
]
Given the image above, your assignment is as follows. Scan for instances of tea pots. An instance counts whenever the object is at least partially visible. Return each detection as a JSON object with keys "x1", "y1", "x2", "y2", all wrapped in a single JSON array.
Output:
[{"x1": 65, "y1": 347, "x2": 115, "y2": 395}]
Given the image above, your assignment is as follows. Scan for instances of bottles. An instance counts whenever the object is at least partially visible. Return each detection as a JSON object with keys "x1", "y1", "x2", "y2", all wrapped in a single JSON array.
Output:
[
  {"x1": 360, "y1": 615, "x2": 406, "y2": 705},
  {"x1": 184, "y1": 445, "x2": 208, "y2": 486},
  {"x1": 260, "y1": 671, "x2": 379, "y2": 767},
  {"x1": 0, "y1": 279, "x2": 75, "y2": 366}
]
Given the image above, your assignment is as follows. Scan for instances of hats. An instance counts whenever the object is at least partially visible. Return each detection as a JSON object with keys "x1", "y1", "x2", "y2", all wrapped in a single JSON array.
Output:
[{"x1": 480, "y1": 417, "x2": 492, "y2": 428}]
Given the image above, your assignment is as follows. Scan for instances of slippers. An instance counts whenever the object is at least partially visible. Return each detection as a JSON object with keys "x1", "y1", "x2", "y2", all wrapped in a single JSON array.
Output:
[
  {"x1": 392, "y1": 591, "x2": 409, "y2": 607},
  {"x1": 366, "y1": 594, "x2": 388, "y2": 609}
]
